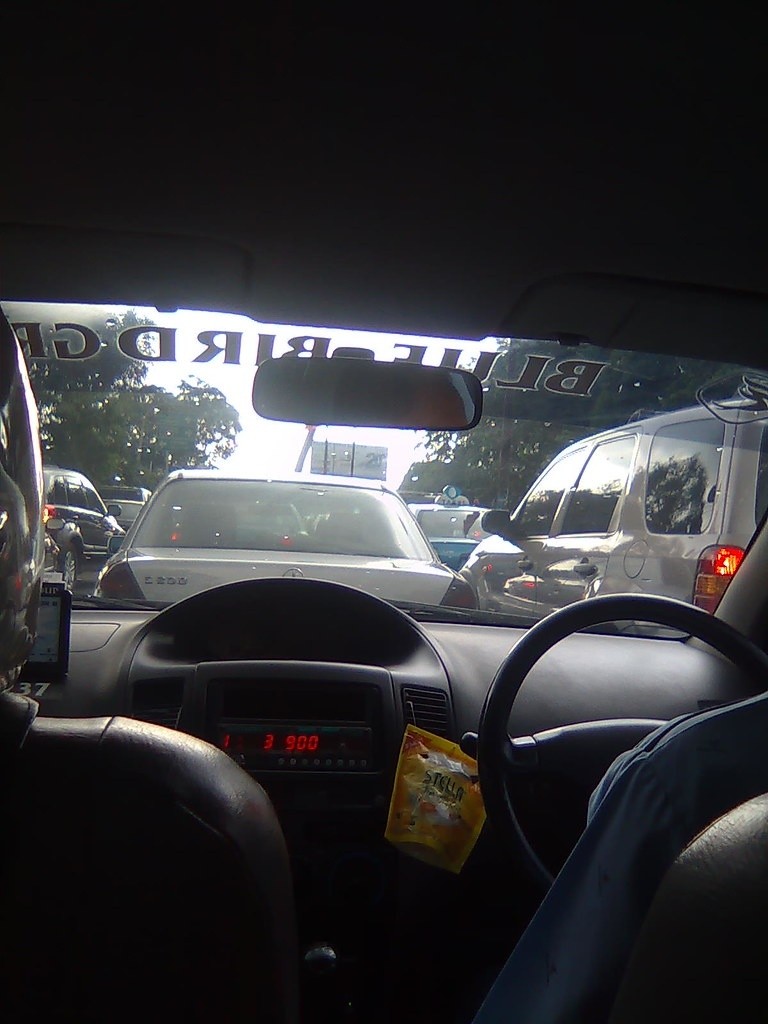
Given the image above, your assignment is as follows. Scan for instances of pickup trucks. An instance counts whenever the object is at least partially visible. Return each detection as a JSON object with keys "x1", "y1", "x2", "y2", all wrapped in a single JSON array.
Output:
[{"x1": 98, "y1": 485, "x2": 154, "y2": 533}]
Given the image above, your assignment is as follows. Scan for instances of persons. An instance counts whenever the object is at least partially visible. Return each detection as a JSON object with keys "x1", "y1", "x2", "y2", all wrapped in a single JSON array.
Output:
[{"x1": 470, "y1": 691, "x2": 768, "y2": 1024}]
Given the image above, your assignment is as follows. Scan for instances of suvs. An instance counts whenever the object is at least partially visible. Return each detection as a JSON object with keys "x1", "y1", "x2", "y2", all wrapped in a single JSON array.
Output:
[
  {"x1": 463, "y1": 400, "x2": 768, "y2": 636},
  {"x1": 35, "y1": 465, "x2": 131, "y2": 583}
]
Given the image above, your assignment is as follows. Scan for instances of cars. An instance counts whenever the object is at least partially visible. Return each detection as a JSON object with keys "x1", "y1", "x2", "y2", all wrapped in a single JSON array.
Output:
[
  {"x1": 89, "y1": 466, "x2": 481, "y2": 617},
  {"x1": 406, "y1": 505, "x2": 496, "y2": 571},
  {"x1": 235, "y1": 499, "x2": 334, "y2": 554}
]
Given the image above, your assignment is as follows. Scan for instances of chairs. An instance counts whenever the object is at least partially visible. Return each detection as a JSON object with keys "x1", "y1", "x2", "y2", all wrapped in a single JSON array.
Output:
[
  {"x1": 602, "y1": 792, "x2": 768, "y2": 1024},
  {"x1": 1, "y1": 694, "x2": 299, "y2": 1024}
]
